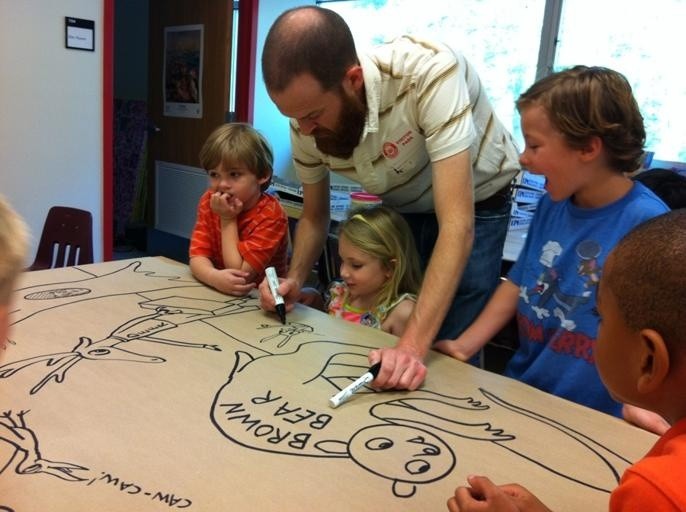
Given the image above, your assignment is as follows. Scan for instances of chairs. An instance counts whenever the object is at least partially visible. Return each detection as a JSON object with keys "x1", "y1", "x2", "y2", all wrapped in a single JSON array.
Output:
[{"x1": 18, "y1": 205, "x2": 95, "y2": 272}]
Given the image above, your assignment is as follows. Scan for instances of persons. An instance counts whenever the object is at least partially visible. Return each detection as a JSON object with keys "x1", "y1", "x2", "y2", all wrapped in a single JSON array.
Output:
[
  {"x1": 430, "y1": 63, "x2": 673, "y2": 436},
  {"x1": 296, "y1": 204, "x2": 425, "y2": 338},
  {"x1": 0, "y1": 199, "x2": 34, "y2": 363},
  {"x1": 186, "y1": 122, "x2": 290, "y2": 299},
  {"x1": 257, "y1": 5, "x2": 523, "y2": 397},
  {"x1": 445, "y1": 205, "x2": 686, "y2": 512}
]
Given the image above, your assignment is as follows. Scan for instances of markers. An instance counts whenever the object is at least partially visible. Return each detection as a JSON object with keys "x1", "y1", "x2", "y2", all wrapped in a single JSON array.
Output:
[
  {"x1": 329, "y1": 364, "x2": 381, "y2": 408},
  {"x1": 264, "y1": 265, "x2": 286, "y2": 326}
]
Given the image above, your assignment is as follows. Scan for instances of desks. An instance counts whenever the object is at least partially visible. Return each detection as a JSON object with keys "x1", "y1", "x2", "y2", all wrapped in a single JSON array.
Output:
[{"x1": 0, "y1": 256, "x2": 663, "y2": 512}]
